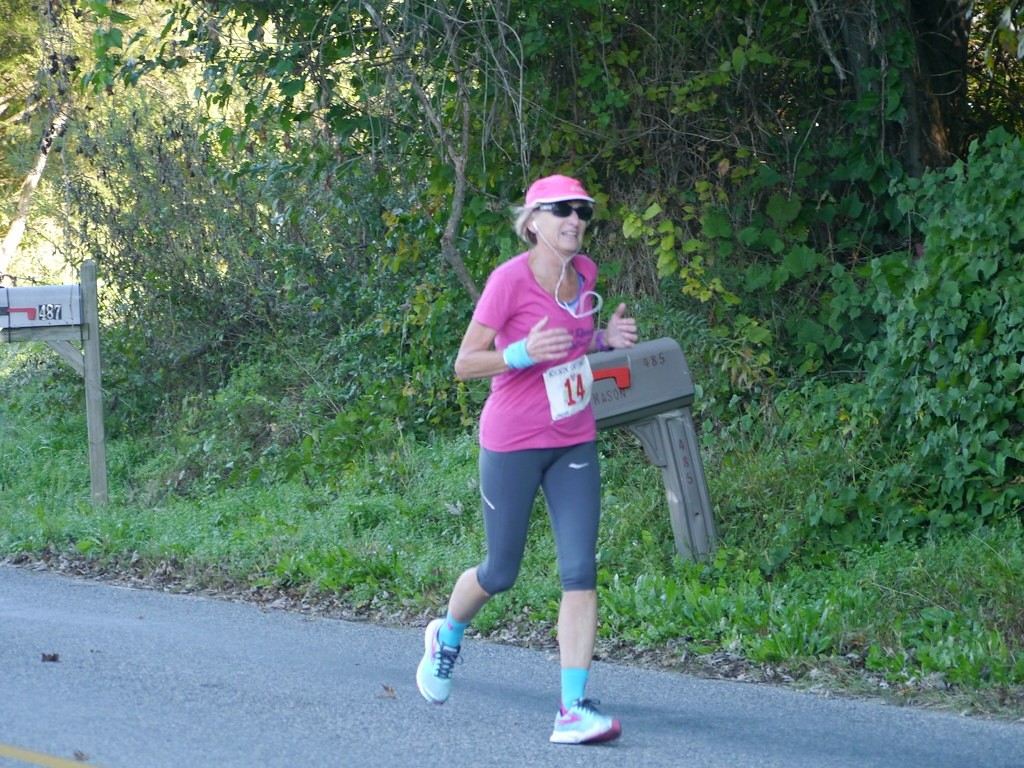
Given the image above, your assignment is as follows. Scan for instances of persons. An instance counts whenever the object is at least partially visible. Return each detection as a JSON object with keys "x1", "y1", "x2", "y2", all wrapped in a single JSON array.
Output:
[{"x1": 414, "y1": 173, "x2": 639, "y2": 744}]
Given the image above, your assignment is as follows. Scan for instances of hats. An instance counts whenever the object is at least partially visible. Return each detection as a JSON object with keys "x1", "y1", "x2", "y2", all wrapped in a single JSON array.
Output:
[{"x1": 525, "y1": 175, "x2": 595, "y2": 208}]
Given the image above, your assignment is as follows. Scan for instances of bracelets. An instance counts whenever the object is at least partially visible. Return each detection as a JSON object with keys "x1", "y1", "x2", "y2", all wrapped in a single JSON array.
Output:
[
  {"x1": 503, "y1": 337, "x2": 535, "y2": 370},
  {"x1": 595, "y1": 330, "x2": 614, "y2": 352}
]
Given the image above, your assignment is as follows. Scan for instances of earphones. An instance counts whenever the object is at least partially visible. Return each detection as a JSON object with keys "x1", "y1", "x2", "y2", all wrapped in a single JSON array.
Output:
[{"x1": 531, "y1": 220, "x2": 539, "y2": 232}]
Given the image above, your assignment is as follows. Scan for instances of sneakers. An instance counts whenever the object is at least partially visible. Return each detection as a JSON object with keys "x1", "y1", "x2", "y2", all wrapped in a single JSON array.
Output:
[
  {"x1": 550, "y1": 697, "x2": 621, "y2": 744},
  {"x1": 416, "y1": 619, "x2": 464, "y2": 705}
]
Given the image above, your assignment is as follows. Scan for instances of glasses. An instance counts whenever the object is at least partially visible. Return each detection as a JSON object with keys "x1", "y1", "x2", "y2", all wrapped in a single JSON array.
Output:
[{"x1": 534, "y1": 202, "x2": 593, "y2": 221}]
